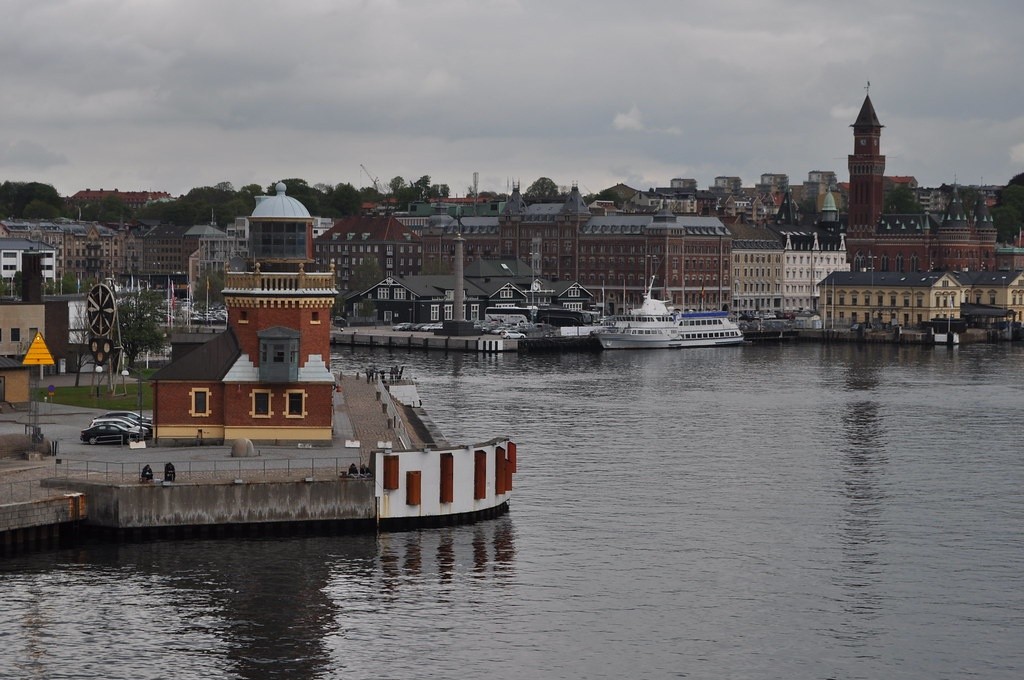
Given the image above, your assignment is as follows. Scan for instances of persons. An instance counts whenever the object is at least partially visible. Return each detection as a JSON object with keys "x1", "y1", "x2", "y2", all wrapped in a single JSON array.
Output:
[
  {"x1": 141, "y1": 464, "x2": 154, "y2": 482},
  {"x1": 359, "y1": 463, "x2": 370, "y2": 477},
  {"x1": 389, "y1": 365, "x2": 400, "y2": 384},
  {"x1": 164, "y1": 462, "x2": 175, "y2": 482},
  {"x1": 348, "y1": 463, "x2": 360, "y2": 478}
]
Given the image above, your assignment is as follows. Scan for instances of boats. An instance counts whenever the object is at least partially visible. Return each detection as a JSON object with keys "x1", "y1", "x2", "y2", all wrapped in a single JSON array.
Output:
[{"x1": 591, "y1": 283, "x2": 744, "y2": 349}]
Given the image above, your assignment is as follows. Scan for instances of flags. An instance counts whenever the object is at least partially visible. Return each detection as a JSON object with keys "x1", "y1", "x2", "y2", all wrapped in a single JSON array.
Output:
[{"x1": 172, "y1": 281, "x2": 175, "y2": 311}]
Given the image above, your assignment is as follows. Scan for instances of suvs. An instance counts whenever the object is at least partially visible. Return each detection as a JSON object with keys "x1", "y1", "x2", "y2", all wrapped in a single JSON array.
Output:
[
  {"x1": 332, "y1": 316, "x2": 347, "y2": 326},
  {"x1": 91, "y1": 419, "x2": 148, "y2": 437},
  {"x1": 501, "y1": 329, "x2": 526, "y2": 339}
]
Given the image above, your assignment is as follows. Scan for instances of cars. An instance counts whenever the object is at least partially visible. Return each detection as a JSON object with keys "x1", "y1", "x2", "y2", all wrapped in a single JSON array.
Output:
[
  {"x1": 391, "y1": 322, "x2": 442, "y2": 332},
  {"x1": 474, "y1": 319, "x2": 532, "y2": 334},
  {"x1": 88, "y1": 412, "x2": 151, "y2": 424},
  {"x1": 157, "y1": 297, "x2": 227, "y2": 325},
  {"x1": 729, "y1": 307, "x2": 795, "y2": 320},
  {"x1": 850, "y1": 324, "x2": 866, "y2": 330},
  {"x1": 107, "y1": 416, "x2": 151, "y2": 428},
  {"x1": 80, "y1": 424, "x2": 145, "y2": 445}
]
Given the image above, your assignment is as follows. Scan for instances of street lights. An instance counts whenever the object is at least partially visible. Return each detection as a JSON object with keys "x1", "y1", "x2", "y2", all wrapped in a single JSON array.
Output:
[{"x1": 95, "y1": 366, "x2": 103, "y2": 397}]
